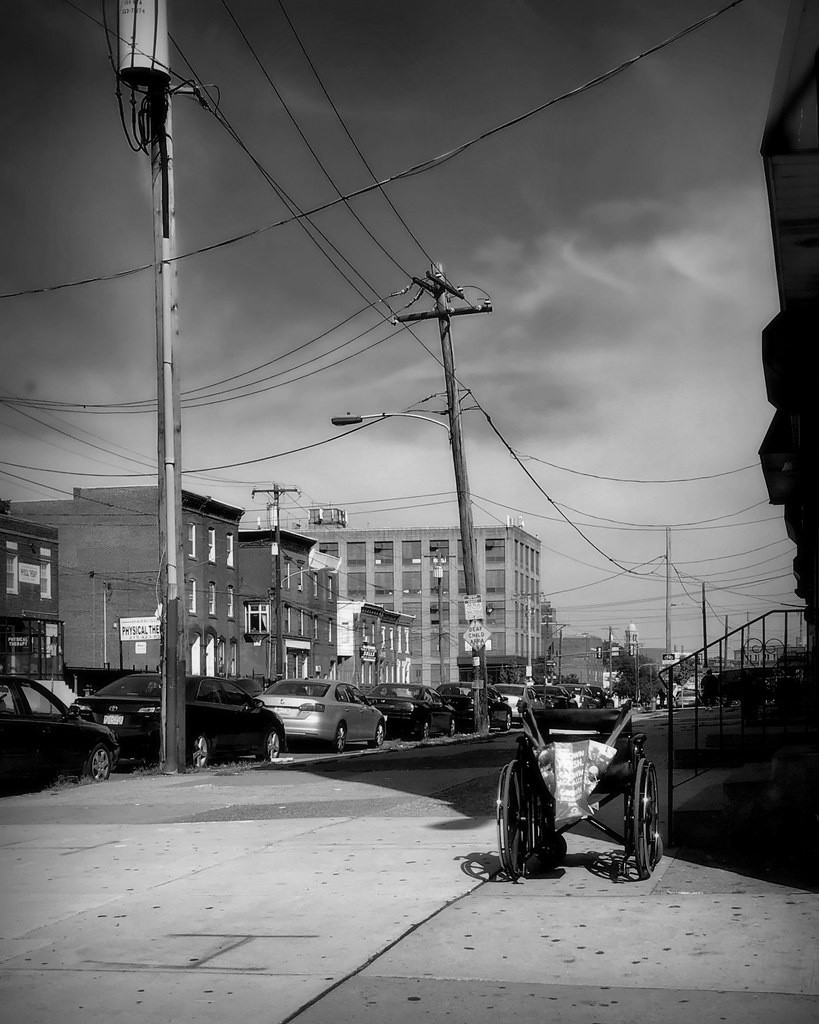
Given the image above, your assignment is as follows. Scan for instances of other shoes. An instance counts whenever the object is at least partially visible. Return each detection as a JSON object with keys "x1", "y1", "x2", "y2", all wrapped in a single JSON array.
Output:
[{"x1": 703, "y1": 705, "x2": 715, "y2": 712}]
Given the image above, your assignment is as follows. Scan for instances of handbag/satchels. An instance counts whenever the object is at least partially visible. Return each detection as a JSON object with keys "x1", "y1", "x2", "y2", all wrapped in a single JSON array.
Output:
[{"x1": 524, "y1": 709, "x2": 633, "y2": 823}]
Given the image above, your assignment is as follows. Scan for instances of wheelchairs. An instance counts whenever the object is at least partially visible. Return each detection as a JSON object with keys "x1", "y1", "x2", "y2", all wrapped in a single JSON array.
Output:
[{"x1": 495, "y1": 699, "x2": 663, "y2": 882}]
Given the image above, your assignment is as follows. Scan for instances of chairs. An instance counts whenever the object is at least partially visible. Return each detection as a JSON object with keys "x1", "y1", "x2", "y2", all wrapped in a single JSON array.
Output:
[
  {"x1": 295, "y1": 688, "x2": 308, "y2": 695},
  {"x1": 386, "y1": 692, "x2": 397, "y2": 698},
  {"x1": 450, "y1": 688, "x2": 460, "y2": 696}
]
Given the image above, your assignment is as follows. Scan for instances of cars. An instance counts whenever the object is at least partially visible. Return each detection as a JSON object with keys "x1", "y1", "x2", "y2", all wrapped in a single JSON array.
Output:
[
  {"x1": 492, "y1": 683, "x2": 544, "y2": 723},
  {"x1": 0, "y1": 674, "x2": 121, "y2": 794},
  {"x1": 588, "y1": 686, "x2": 615, "y2": 710},
  {"x1": 555, "y1": 684, "x2": 602, "y2": 709},
  {"x1": 435, "y1": 681, "x2": 513, "y2": 732},
  {"x1": 250, "y1": 676, "x2": 388, "y2": 755},
  {"x1": 362, "y1": 682, "x2": 458, "y2": 741},
  {"x1": 532, "y1": 685, "x2": 579, "y2": 710},
  {"x1": 674, "y1": 690, "x2": 697, "y2": 707},
  {"x1": 69, "y1": 671, "x2": 287, "y2": 769}
]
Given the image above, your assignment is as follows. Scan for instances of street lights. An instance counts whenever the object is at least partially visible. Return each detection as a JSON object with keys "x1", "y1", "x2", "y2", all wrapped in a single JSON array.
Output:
[
  {"x1": 330, "y1": 411, "x2": 492, "y2": 739},
  {"x1": 275, "y1": 567, "x2": 335, "y2": 681},
  {"x1": 670, "y1": 602, "x2": 707, "y2": 669},
  {"x1": 433, "y1": 550, "x2": 450, "y2": 681}
]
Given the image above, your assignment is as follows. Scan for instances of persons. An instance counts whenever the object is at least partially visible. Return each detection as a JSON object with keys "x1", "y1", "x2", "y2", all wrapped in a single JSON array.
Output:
[
  {"x1": 701, "y1": 670, "x2": 719, "y2": 712},
  {"x1": 658, "y1": 689, "x2": 665, "y2": 711}
]
